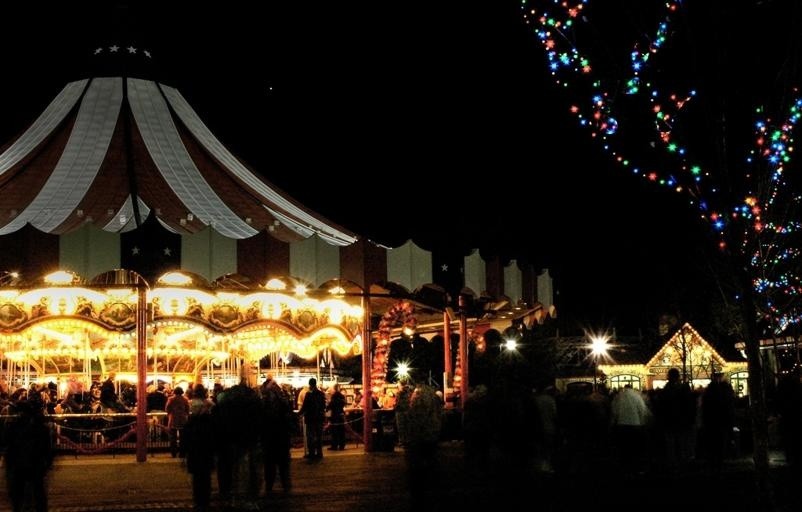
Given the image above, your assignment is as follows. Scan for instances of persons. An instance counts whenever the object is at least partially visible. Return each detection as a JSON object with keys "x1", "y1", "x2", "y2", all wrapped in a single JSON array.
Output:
[
  {"x1": 612, "y1": 368, "x2": 736, "y2": 430},
  {"x1": 325, "y1": 383, "x2": 345, "y2": 451},
  {"x1": 0, "y1": 371, "x2": 164, "y2": 415},
  {"x1": 0, "y1": 396, "x2": 57, "y2": 512},
  {"x1": 293, "y1": 378, "x2": 326, "y2": 460},
  {"x1": 393, "y1": 377, "x2": 442, "y2": 453},
  {"x1": 164, "y1": 375, "x2": 293, "y2": 512},
  {"x1": 340, "y1": 388, "x2": 381, "y2": 410}
]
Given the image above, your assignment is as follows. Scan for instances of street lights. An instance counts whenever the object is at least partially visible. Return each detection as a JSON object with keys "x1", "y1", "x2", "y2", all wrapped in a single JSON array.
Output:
[{"x1": 593, "y1": 339, "x2": 603, "y2": 394}]
